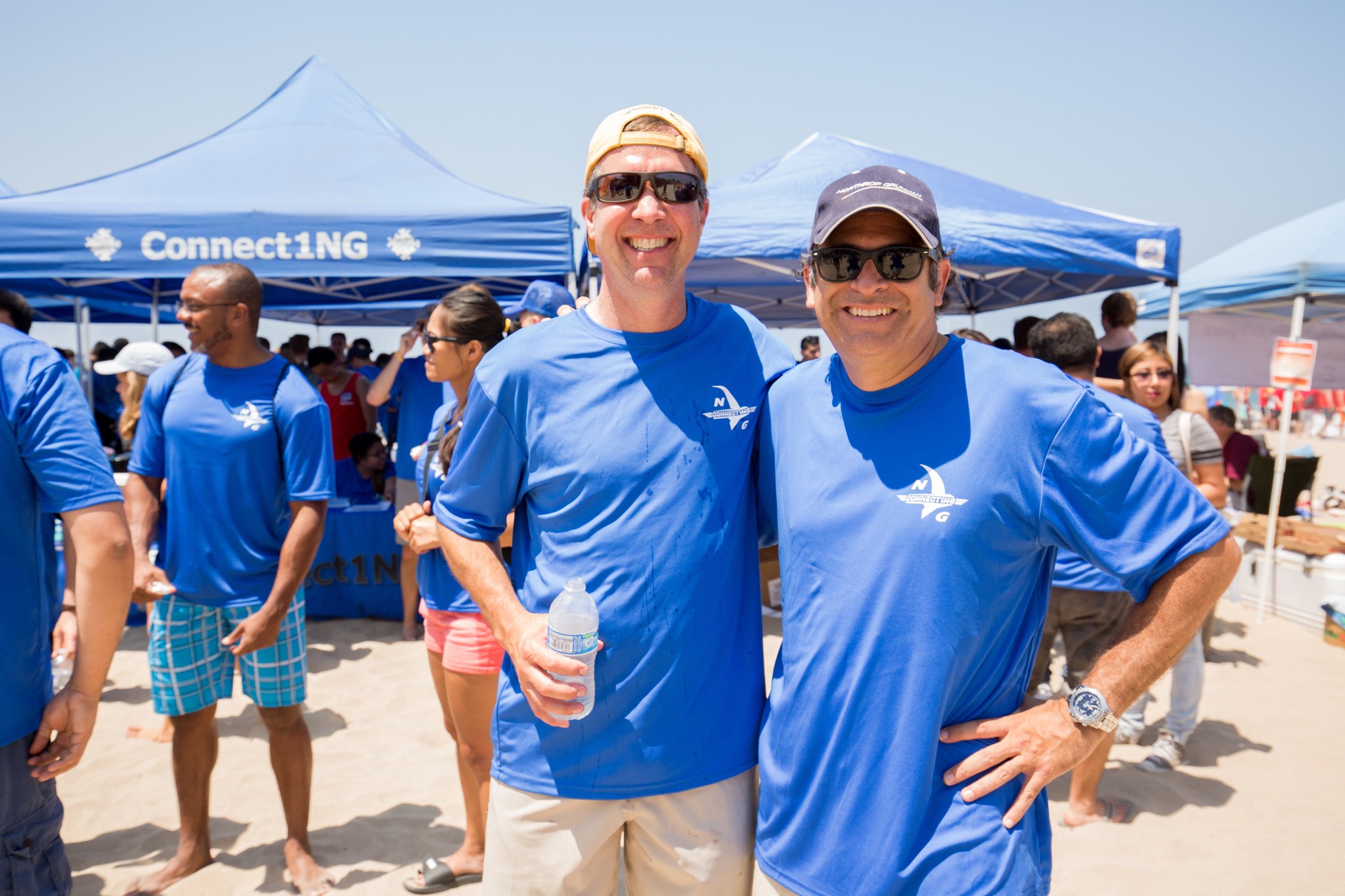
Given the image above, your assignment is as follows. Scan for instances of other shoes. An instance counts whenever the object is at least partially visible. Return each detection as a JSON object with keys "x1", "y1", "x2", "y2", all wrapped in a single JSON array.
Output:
[
  {"x1": 1137, "y1": 728, "x2": 1186, "y2": 772},
  {"x1": 1114, "y1": 729, "x2": 1141, "y2": 745}
]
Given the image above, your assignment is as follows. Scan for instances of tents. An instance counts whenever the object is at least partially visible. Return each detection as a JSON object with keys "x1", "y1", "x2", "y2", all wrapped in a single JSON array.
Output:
[
  {"x1": 580, "y1": 127, "x2": 1185, "y2": 385},
  {"x1": 0, "y1": 51, "x2": 579, "y2": 420},
  {"x1": 1135, "y1": 201, "x2": 1345, "y2": 623}
]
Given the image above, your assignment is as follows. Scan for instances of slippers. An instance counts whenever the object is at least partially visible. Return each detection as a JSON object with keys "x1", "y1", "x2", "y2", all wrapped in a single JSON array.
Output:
[{"x1": 403, "y1": 857, "x2": 483, "y2": 894}]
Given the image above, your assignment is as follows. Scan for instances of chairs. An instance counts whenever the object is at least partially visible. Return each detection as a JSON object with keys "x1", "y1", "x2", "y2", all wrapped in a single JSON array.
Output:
[{"x1": 1245, "y1": 453, "x2": 1321, "y2": 520}]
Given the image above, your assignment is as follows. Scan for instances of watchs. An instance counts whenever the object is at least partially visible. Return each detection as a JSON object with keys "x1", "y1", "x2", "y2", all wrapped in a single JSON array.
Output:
[{"x1": 1067, "y1": 684, "x2": 1120, "y2": 733}]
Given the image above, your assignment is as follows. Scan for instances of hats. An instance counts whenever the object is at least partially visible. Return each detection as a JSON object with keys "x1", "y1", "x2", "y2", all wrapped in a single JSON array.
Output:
[
  {"x1": 354, "y1": 338, "x2": 373, "y2": 353},
  {"x1": 583, "y1": 104, "x2": 708, "y2": 188},
  {"x1": 809, "y1": 165, "x2": 946, "y2": 250},
  {"x1": 93, "y1": 342, "x2": 175, "y2": 376},
  {"x1": 502, "y1": 279, "x2": 577, "y2": 319}
]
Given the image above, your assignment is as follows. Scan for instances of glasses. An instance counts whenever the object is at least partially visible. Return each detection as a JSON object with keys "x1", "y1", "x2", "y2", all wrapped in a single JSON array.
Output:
[
  {"x1": 182, "y1": 300, "x2": 236, "y2": 314},
  {"x1": 424, "y1": 326, "x2": 471, "y2": 354},
  {"x1": 368, "y1": 448, "x2": 386, "y2": 458},
  {"x1": 586, "y1": 170, "x2": 707, "y2": 204},
  {"x1": 810, "y1": 245, "x2": 940, "y2": 284},
  {"x1": 1130, "y1": 369, "x2": 1172, "y2": 380}
]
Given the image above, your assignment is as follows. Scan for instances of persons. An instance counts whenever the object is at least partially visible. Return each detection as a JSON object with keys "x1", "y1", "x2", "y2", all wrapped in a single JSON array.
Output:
[
  {"x1": 365, "y1": 303, "x2": 445, "y2": 641},
  {"x1": 255, "y1": 331, "x2": 395, "y2": 508},
  {"x1": 941, "y1": 288, "x2": 1261, "y2": 828},
  {"x1": 435, "y1": 107, "x2": 829, "y2": 896},
  {"x1": 797, "y1": 335, "x2": 821, "y2": 363},
  {"x1": 0, "y1": 285, "x2": 194, "y2": 745},
  {"x1": 0, "y1": 321, "x2": 135, "y2": 896},
  {"x1": 394, "y1": 282, "x2": 519, "y2": 895},
  {"x1": 120, "y1": 263, "x2": 342, "y2": 896},
  {"x1": 756, "y1": 166, "x2": 1243, "y2": 896},
  {"x1": 500, "y1": 279, "x2": 578, "y2": 341}
]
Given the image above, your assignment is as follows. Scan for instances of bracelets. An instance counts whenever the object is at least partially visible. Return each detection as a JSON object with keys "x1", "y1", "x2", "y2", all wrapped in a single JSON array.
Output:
[{"x1": 395, "y1": 351, "x2": 404, "y2": 362}]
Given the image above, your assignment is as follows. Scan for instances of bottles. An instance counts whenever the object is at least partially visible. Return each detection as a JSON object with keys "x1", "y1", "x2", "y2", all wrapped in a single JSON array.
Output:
[
  {"x1": 51, "y1": 649, "x2": 73, "y2": 695},
  {"x1": 547, "y1": 577, "x2": 600, "y2": 720}
]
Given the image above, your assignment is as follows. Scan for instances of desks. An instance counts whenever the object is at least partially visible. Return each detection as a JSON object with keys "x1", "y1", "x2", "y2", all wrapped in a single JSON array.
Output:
[{"x1": 305, "y1": 499, "x2": 423, "y2": 621}]
